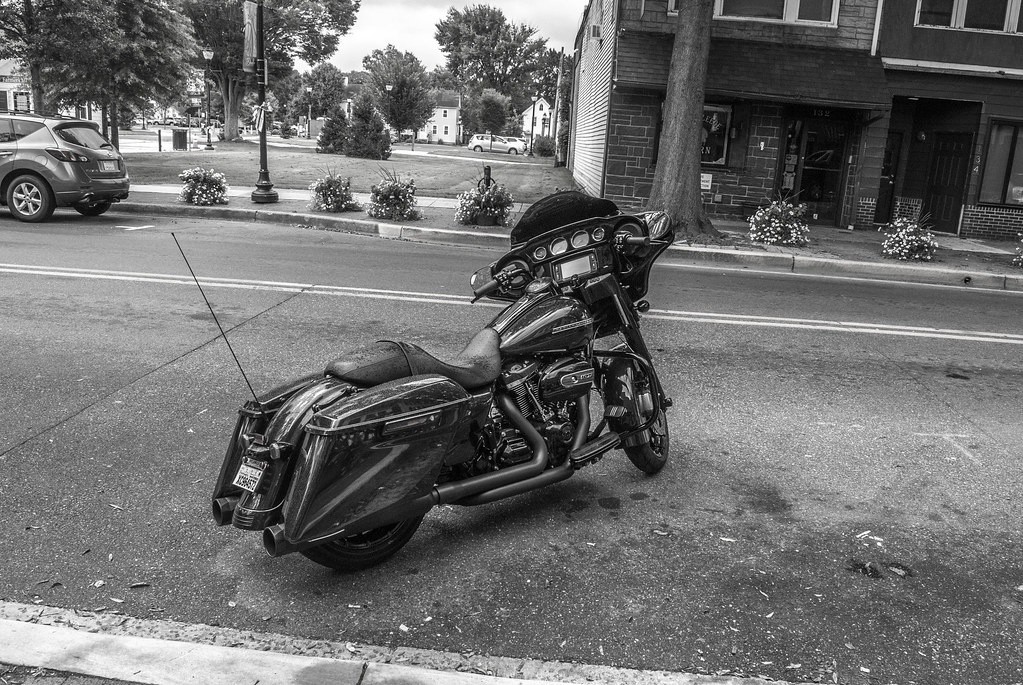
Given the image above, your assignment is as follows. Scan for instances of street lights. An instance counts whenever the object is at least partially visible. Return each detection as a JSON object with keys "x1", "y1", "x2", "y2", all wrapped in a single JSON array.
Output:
[
  {"x1": 386, "y1": 79, "x2": 393, "y2": 125},
  {"x1": 527, "y1": 92, "x2": 538, "y2": 157},
  {"x1": 202, "y1": 42, "x2": 215, "y2": 150},
  {"x1": 306, "y1": 85, "x2": 313, "y2": 139}
]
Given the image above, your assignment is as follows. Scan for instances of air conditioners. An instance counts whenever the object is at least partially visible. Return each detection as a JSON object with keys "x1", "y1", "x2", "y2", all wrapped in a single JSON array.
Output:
[{"x1": 590, "y1": 24, "x2": 603, "y2": 40}]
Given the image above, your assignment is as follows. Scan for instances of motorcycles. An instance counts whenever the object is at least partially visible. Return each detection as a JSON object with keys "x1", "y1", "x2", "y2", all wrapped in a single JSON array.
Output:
[{"x1": 210, "y1": 190, "x2": 672, "y2": 573}]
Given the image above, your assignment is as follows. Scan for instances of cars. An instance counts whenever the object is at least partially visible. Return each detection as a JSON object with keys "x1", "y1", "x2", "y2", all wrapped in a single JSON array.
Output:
[{"x1": 130, "y1": 115, "x2": 204, "y2": 128}]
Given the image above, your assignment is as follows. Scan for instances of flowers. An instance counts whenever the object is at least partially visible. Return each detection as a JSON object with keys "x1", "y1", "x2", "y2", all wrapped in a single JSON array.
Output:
[
  {"x1": 877, "y1": 203, "x2": 939, "y2": 263},
  {"x1": 176, "y1": 167, "x2": 230, "y2": 206},
  {"x1": 454, "y1": 168, "x2": 516, "y2": 229},
  {"x1": 1011, "y1": 233, "x2": 1023, "y2": 269},
  {"x1": 366, "y1": 163, "x2": 427, "y2": 220},
  {"x1": 305, "y1": 165, "x2": 364, "y2": 212},
  {"x1": 747, "y1": 189, "x2": 810, "y2": 247}
]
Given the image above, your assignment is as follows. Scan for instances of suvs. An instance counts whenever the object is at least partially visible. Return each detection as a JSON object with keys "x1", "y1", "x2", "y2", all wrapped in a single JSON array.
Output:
[
  {"x1": 503, "y1": 136, "x2": 527, "y2": 151},
  {"x1": 0, "y1": 108, "x2": 131, "y2": 223},
  {"x1": 468, "y1": 133, "x2": 524, "y2": 155}
]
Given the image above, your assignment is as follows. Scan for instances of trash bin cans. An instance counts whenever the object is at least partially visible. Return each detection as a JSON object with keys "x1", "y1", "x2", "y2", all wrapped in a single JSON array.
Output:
[{"x1": 171, "y1": 128, "x2": 188, "y2": 151}]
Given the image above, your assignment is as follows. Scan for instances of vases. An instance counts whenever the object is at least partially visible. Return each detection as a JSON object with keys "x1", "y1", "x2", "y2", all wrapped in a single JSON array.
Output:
[{"x1": 475, "y1": 215, "x2": 497, "y2": 226}]
